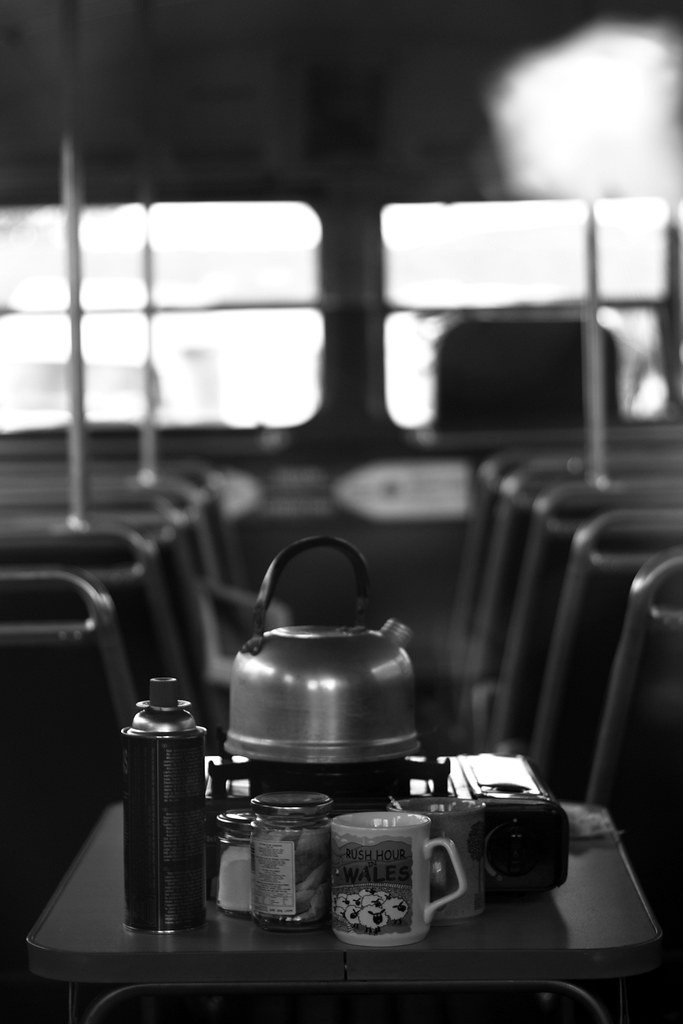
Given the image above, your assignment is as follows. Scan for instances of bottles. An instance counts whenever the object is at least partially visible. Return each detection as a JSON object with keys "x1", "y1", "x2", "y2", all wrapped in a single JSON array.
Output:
[
  {"x1": 117, "y1": 675, "x2": 208, "y2": 933},
  {"x1": 212, "y1": 810, "x2": 252, "y2": 917},
  {"x1": 252, "y1": 793, "x2": 333, "y2": 931}
]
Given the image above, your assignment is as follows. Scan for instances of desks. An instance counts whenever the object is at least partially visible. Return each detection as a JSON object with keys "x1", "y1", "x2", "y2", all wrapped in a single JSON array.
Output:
[{"x1": 20, "y1": 794, "x2": 663, "y2": 1024}]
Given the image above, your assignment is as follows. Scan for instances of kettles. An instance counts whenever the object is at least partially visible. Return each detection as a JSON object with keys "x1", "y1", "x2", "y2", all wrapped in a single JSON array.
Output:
[{"x1": 222, "y1": 535, "x2": 421, "y2": 762}]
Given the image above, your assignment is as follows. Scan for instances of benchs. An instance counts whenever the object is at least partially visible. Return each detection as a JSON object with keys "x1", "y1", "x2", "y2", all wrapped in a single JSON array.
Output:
[
  {"x1": 448, "y1": 453, "x2": 683, "y2": 1024},
  {"x1": 0, "y1": 459, "x2": 246, "y2": 1024}
]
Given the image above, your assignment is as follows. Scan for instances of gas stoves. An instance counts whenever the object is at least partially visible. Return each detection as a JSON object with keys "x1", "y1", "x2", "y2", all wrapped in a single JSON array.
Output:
[{"x1": 205, "y1": 752, "x2": 570, "y2": 901}]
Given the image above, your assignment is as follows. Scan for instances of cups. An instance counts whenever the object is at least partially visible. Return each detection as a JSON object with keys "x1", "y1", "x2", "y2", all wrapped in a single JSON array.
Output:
[{"x1": 329, "y1": 810, "x2": 468, "y2": 948}]
